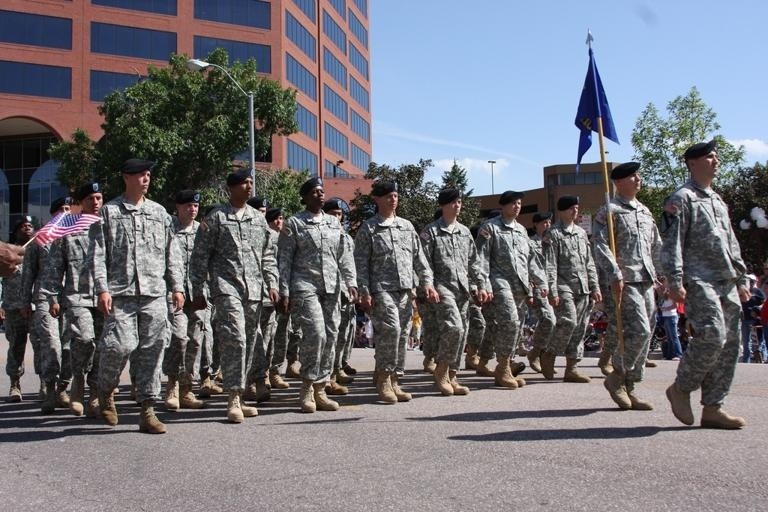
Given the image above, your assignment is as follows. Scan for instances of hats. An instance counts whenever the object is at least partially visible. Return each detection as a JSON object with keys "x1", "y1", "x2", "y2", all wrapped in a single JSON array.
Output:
[
  {"x1": 499, "y1": 190, "x2": 524, "y2": 206},
  {"x1": 684, "y1": 139, "x2": 718, "y2": 160},
  {"x1": 532, "y1": 212, "x2": 553, "y2": 223},
  {"x1": 556, "y1": 195, "x2": 580, "y2": 211},
  {"x1": 469, "y1": 210, "x2": 502, "y2": 240},
  {"x1": 322, "y1": 200, "x2": 342, "y2": 213},
  {"x1": 370, "y1": 183, "x2": 398, "y2": 196},
  {"x1": 609, "y1": 162, "x2": 641, "y2": 180},
  {"x1": 175, "y1": 189, "x2": 201, "y2": 205},
  {"x1": 226, "y1": 168, "x2": 252, "y2": 187},
  {"x1": 434, "y1": 210, "x2": 443, "y2": 220},
  {"x1": 76, "y1": 183, "x2": 103, "y2": 200},
  {"x1": 298, "y1": 176, "x2": 324, "y2": 199},
  {"x1": 438, "y1": 188, "x2": 459, "y2": 206},
  {"x1": 50, "y1": 196, "x2": 75, "y2": 215},
  {"x1": 266, "y1": 207, "x2": 282, "y2": 221},
  {"x1": 15, "y1": 215, "x2": 33, "y2": 230},
  {"x1": 121, "y1": 159, "x2": 154, "y2": 175},
  {"x1": 251, "y1": 198, "x2": 266, "y2": 209}
]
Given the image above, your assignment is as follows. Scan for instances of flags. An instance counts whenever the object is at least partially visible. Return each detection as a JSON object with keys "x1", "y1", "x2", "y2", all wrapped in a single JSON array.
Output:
[{"x1": 575, "y1": 48, "x2": 620, "y2": 175}]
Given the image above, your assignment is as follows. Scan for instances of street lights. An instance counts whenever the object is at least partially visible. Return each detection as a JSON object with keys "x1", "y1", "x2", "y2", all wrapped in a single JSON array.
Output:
[
  {"x1": 738, "y1": 206, "x2": 767, "y2": 258},
  {"x1": 332, "y1": 158, "x2": 344, "y2": 178},
  {"x1": 186, "y1": 58, "x2": 258, "y2": 201},
  {"x1": 486, "y1": 160, "x2": 497, "y2": 194}
]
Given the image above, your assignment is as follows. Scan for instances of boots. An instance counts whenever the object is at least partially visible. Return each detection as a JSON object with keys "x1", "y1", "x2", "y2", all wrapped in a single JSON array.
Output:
[
  {"x1": 373, "y1": 343, "x2": 591, "y2": 403},
  {"x1": 36, "y1": 361, "x2": 119, "y2": 426},
  {"x1": 665, "y1": 382, "x2": 694, "y2": 425},
  {"x1": 700, "y1": 402, "x2": 746, "y2": 429},
  {"x1": 229, "y1": 362, "x2": 299, "y2": 423},
  {"x1": 8, "y1": 379, "x2": 22, "y2": 402},
  {"x1": 597, "y1": 356, "x2": 656, "y2": 411},
  {"x1": 131, "y1": 377, "x2": 223, "y2": 433},
  {"x1": 299, "y1": 365, "x2": 356, "y2": 412}
]
